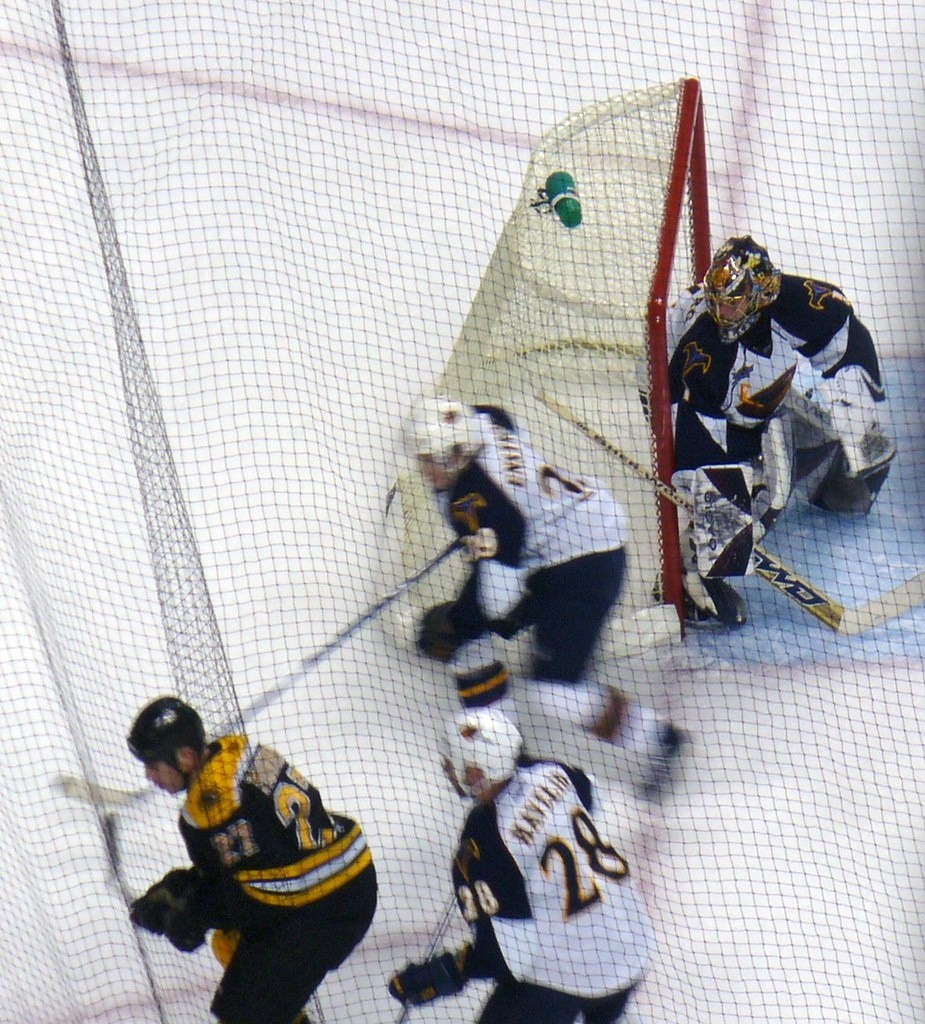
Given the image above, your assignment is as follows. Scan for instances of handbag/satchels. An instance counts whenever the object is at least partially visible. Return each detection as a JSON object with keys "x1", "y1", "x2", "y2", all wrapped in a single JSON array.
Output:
[{"x1": 416, "y1": 599, "x2": 470, "y2": 664}]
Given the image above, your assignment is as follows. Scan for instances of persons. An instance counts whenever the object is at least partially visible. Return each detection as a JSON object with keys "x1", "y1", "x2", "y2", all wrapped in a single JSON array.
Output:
[
  {"x1": 125, "y1": 696, "x2": 378, "y2": 1024},
  {"x1": 388, "y1": 705, "x2": 656, "y2": 1024},
  {"x1": 405, "y1": 396, "x2": 688, "y2": 798},
  {"x1": 637, "y1": 236, "x2": 896, "y2": 638}
]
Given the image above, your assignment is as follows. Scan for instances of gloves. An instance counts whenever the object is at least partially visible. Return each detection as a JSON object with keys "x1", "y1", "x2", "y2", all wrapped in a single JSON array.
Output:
[
  {"x1": 387, "y1": 953, "x2": 464, "y2": 1006},
  {"x1": 130, "y1": 870, "x2": 197, "y2": 947},
  {"x1": 490, "y1": 596, "x2": 533, "y2": 639}
]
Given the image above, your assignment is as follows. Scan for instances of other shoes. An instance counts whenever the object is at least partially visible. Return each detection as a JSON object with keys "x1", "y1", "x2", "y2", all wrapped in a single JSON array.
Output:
[
  {"x1": 654, "y1": 578, "x2": 709, "y2": 626},
  {"x1": 635, "y1": 723, "x2": 684, "y2": 789}
]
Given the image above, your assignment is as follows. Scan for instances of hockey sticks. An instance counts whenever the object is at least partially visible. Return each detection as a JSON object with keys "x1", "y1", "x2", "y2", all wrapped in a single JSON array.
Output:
[
  {"x1": 51, "y1": 539, "x2": 466, "y2": 807},
  {"x1": 397, "y1": 890, "x2": 460, "y2": 1024},
  {"x1": 535, "y1": 386, "x2": 925, "y2": 635},
  {"x1": 96, "y1": 811, "x2": 138, "y2": 908}
]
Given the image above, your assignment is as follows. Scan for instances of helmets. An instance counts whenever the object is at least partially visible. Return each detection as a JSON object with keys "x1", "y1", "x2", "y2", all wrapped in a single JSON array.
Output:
[
  {"x1": 713, "y1": 235, "x2": 771, "y2": 273},
  {"x1": 128, "y1": 696, "x2": 207, "y2": 762},
  {"x1": 705, "y1": 254, "x2": 754, "y2": 298},
  {"x1": 413, "y1": 400, "x2": 483, "y2": 456},
  {"x1": 437, "y1": 708, "x2": 521, "y2": 779}
]
